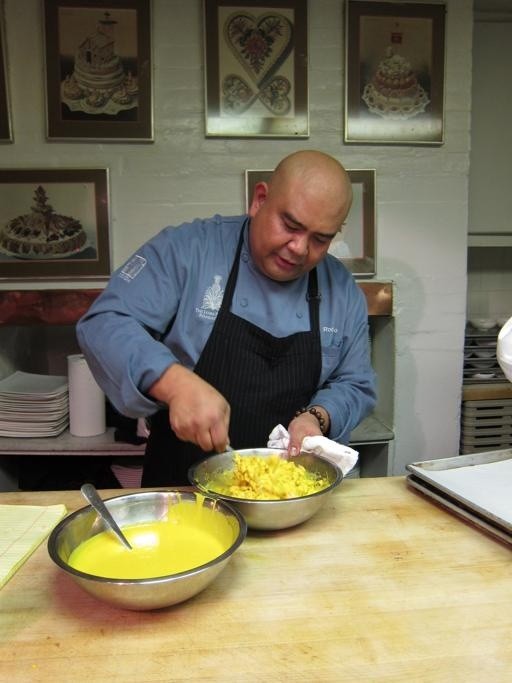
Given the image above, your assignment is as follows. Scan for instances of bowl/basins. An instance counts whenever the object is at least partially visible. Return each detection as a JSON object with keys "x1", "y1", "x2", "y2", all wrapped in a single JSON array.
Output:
[
  {"x1": 497, "y1": 319, "x2": 509, "y2": 327},
  {"x1": 48, "y1": 490, "x2": 247, "y2": 611},
  {"x1": 470, "y1": 319, "x2": 497, "y2": 332},
  {"x1": 188, "y1": 448, "x2": 343, "y2": 531}
]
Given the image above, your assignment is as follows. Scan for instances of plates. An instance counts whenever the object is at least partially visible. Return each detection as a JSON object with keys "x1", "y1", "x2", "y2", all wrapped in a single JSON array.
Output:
[
  {"x1": 405, "y1": 449, "x2": 512, "y2": 538},
  {"x1": 462, "y1": 400, "x2": 512, "y2": 455},
  {"x1": 463, "y1": 339, "x2": 508, "y2": 380},
  {"x1": 0, "y1": 371, "x2": 69, "y2": 438},
  {"x1": 407, "y1": 474, "x2": 512, "y2": 549}
]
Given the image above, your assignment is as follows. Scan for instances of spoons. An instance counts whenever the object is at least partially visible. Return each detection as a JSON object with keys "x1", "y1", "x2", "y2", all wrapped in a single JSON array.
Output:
[
  {"x1": 81, "y1": 484, "x2": 133, "y2": 551},
  {"x1": 225, "y1": 445, "x2": 243, "y2": 467}
]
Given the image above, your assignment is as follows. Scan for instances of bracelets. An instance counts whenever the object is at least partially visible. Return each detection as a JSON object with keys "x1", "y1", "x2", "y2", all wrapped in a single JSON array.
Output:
[{"x1": 295, "y1": 407, "x2": 327, "y2": 436}]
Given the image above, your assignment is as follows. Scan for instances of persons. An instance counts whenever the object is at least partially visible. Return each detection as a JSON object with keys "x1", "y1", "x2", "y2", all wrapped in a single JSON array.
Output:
[{"x1": 76, "y1": 149, "x2": 378, "y2": 488}]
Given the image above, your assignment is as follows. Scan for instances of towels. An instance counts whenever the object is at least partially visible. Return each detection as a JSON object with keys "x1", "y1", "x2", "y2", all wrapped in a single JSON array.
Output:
[{"x1": 267, "y1": 424, "x2": 359, "y2": 480}]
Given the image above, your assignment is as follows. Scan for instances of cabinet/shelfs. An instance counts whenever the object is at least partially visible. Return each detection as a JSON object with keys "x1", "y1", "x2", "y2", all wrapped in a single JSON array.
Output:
[{"x1": 1, "y1": 281, "x2": 396, "y2": 492}]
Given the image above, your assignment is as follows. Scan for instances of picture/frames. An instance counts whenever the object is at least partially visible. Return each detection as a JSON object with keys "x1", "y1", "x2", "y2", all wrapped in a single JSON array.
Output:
[
  {"x1": 1, "y1": 169, "x2": 111, "y2": 277},
  {"x1": 44, "y1": 0, "x2": 154, "y2": 142},
  {"x1": 203, "y1": 1, "x2": 309, "y2": 137},
  {"x1": 245, "y1": 169, "x2": 376, "y2": 275},
  {"x1": 344, "y1": 0, "x2": 446, "y2": 145}
]
